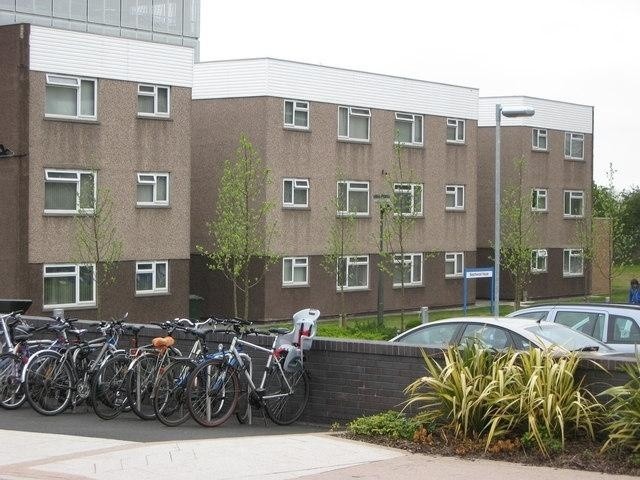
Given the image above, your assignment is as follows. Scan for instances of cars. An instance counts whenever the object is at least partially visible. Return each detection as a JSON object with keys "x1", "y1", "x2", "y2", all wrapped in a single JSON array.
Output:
[{"x1": 387, "y1": 299, "x2": 640, "y2": 367}]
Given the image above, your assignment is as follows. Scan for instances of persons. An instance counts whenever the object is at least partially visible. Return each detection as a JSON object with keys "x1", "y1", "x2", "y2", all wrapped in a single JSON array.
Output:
[{"x1": 629, "y1": 278, "x2": 640, "y2": 306}]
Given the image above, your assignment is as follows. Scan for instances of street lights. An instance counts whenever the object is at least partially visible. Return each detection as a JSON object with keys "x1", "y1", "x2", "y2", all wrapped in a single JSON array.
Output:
[{"x1": 491, "y1": 102, "x2": 536, "y2": 323}]
